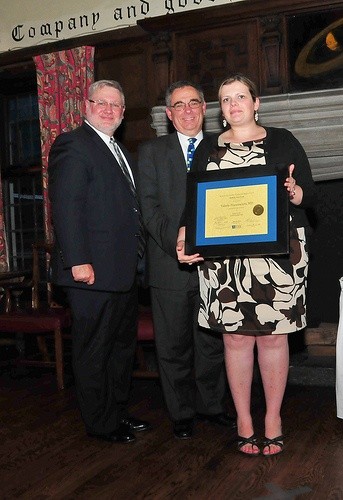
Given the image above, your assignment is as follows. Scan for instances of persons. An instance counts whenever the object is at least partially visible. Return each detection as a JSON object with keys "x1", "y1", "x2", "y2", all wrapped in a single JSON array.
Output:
[
  {"x1": 176, "y1": 75, "x2": 316, "y2": 459},
  {"x1": 46, "y1": 77, "x2": 160, "y2": 445},
  {"x1": 132, "y1": 80, "x2": 240, "y2": 441}
]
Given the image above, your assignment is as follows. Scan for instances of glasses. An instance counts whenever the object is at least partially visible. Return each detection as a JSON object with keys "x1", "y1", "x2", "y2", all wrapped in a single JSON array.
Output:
[
  {"x1": 167, "y1": 98, "x2": 202, "y2": 111},
  {"x1": 88, "y1": 98, "x2": 125, "y2": 112}
]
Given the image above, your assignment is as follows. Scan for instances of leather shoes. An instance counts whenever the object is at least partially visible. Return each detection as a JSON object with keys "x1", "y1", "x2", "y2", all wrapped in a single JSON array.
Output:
[
  {"x1": 197, "y1": 411, "x2": 237, "y2": 429},
  {"x1": 173, "y1": 416, "x2": 196, "y2": 440},
  {"x1": 122, "y1": 415, "x2": 153, "y2": 432},
  {"x1": 87, "y1": 429, "x2": 136, "y2": 444}
]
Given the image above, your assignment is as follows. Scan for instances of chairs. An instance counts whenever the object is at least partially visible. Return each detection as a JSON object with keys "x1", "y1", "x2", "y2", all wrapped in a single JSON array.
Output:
[{"x1": 0, "y1": 243, "x2": 73, "y2": 393}]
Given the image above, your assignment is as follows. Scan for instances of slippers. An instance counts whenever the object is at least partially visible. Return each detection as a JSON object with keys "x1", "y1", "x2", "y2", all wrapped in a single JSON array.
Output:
[
  {"x1": 235, "y1": 425, "x2": 262, "y2": 458},
  {"x1": 261, "y1": 434, "x2": 285, "y2": 457}
]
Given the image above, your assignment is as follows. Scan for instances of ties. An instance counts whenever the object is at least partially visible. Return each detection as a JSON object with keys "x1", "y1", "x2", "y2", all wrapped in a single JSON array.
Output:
[
  {"x1": 110, "y1": 138, "x2": 133, "y2": 191},
  {"x1": 186, "y1": 138, "x2": 198, "y2": 171}
]
